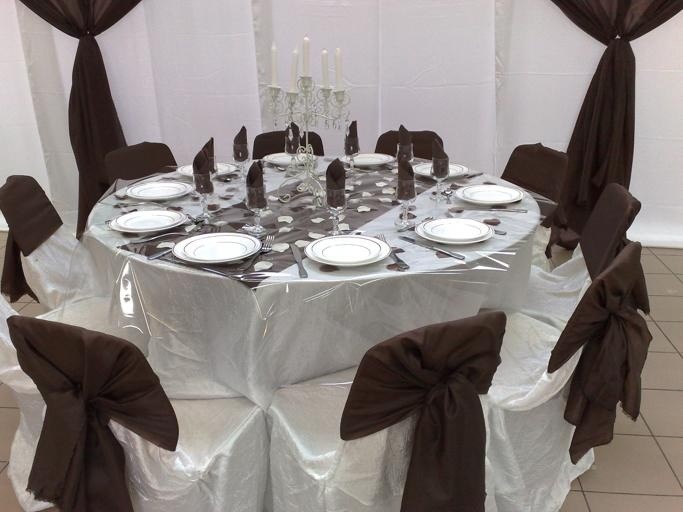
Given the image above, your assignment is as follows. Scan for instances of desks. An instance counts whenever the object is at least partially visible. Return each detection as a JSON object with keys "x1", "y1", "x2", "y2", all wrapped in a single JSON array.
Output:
[{"x1": 88, "y1": 154, "x2": 560, "y2": 413}]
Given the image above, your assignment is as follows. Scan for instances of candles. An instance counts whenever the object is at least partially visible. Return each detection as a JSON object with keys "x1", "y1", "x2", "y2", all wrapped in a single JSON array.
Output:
[
  {"x1": 290, "y1": 47, "x2": 299, "y2": 93},
  {"x1": 321, "y1": 48, "x2": 329, "y2": 89},
  {"x1": 303, "y1": 33, "x2": 310, "y2": 76},
  {"x1": 271, "y1": 45, "x2": 278, "y2": 87},
  {"x1": 334, "y1": 47, "x2": 344, "y2": 92}
]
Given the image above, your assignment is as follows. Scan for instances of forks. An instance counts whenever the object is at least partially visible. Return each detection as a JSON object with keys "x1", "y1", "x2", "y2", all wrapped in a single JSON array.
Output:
[
  {"x1": 131, "y1": 221, "x2": 203, "y2": 244},
  {"x1": 237, "y1": 233, "x2": 274, "y2": 271},
  {"x1": 377, "y1": 232, "x2": 409, "y2": 270}
]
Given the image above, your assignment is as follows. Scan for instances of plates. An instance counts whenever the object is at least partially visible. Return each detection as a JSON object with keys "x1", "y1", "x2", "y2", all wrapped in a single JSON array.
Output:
[
  {"x1": 126, "y1": 182, "x2": 192, "y2": 200},
  {"x1": 413, "y1": 156, "x2": 468, "y2": 181},
  {"x1": 456, "y1": 183, "x2": 525, "y2": 207},
  {"x1": 304, "y1": 234, "x2": 392, "y2": 269},
  {"x1": 108, "y1": 209, "x2": 186, "y2": 235},
  {"x1": 414, "y1": 218, "x2": 494, "y2": 245},
  {"x1": 172, "y1": 231, "x2": 261, "y2": 266}
]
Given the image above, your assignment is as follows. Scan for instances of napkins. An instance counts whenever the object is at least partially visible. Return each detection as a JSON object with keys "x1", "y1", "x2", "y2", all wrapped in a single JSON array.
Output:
[
  {"x1": 344, "y1": 121, "x2": 360, "y2": 157},
  {"x1": 326, "y1": 158, "x2": 347, "y2": 207},
  {"x1": 397, "y1": 159, "x2": 417, "y2": 201},
  {"x1": 284, "y1": 121, "x2": 300, "y2": 155},
  {"x1": 193, "y1": 137, "x2": 215, "y2": 192},
  {"x1": 245, "y1": 160, "x2": 268, "y2": 209},
  {"x1": 429, "y1": 134, "x2": 449, "y2": 180},
  {"x1": 396, "y1": 124, "x2": 413, "y2": 160},
  {"x1": 233, "y1": 126, "x2": 250, "y2": 163}
]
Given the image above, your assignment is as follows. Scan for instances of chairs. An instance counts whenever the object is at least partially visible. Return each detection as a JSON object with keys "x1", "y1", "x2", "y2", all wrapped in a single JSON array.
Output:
[
  {"x1": 1, "y1": 174, "x2": 88, "y2": 309},
  {"x1": 517, "y1": 182, "x2": 642, "y2": 332},
  {"x1": 375, "y1": 130, "x2": 445, "y2": 159},
  {"x1": 266, "y1": 312, "x2": 507, "y2": 512},
  {"x1": 485, "y1": 241, "x2": 642, "y2": 511},
  {"x1": 501, "y1": 142, "x2": 573, "y2": 233},
  {"x1": 251, "y1": 132, "x2": 325, "y2": 160},
  {"x1": 6, "y1": 314, "x2": 268, "y2": 512}
]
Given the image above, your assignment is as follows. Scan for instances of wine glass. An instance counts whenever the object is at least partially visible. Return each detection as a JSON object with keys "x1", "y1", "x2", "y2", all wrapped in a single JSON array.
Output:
[{"x1": 175, "y1": 136, "x2": 449, "y2": 237}]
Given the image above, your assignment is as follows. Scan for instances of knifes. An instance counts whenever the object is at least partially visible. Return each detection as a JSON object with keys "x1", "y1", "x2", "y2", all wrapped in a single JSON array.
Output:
[
  {"x1": 289, "y1": 242, "x2": 308, "y2": 278},
  {"x1": 400, "y1": 235, "x2": 465, "y2": 260},
  {"x1": 456, "y1": 205, "x2": 528, "y2": 214}
]
